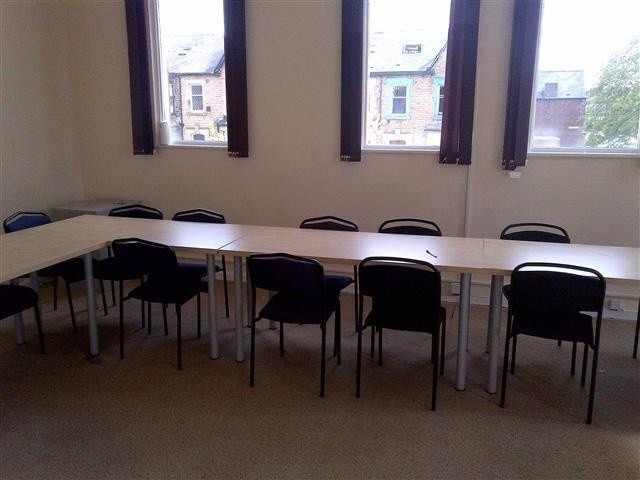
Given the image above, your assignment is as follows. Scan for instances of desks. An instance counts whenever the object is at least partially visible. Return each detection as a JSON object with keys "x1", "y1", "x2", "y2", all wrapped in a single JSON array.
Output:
[
  {"x1": 0, "y1": 215, "x2": 258, "y2": 364},
  {"x1": 484, "y1": 238, "x2": 640, "y2": 394},
  {"x1": 218, "y1": 225, "x2": 484, "y2": 393}
]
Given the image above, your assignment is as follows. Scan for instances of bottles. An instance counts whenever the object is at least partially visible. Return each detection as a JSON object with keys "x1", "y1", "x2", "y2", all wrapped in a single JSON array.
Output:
[{"x1": 158, "y1": 122, "x2": 171, "y2": 145}]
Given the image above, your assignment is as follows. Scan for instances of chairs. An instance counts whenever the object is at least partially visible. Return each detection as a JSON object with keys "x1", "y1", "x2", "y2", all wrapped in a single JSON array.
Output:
[
  {"x1": 370, "y1": 218, "x2": 442, "y2": 363},
  {"x1": 3, "y1": 210, "x2": 108, "y2": 334},
  {"x1": 500, "y1": 223, "x2": 577, "y2": 378},
  {"x1": 94, "y1": 204, "x2": 167, "y2": 328},
  {"x1": 245, "y1": 253, "x2": 341, "y2": 397},
  {"x1": 1, "y1": 284, "x2": 45, "y2": 349},
  {"x1": 354, "y1": 256, "x2": 447, "y2": 411},
  {"x1": 499, "y1": 262, "x2": 606, "y2": 424},
  {"x1": 299, "y1": 216, "x2": 359, "y2": 356},
  {"x1": 148, "y1": 209, "x2": 229, "y2": 338},
  {"x1": 111, "y1": 237, "x2": 208, "y2": 370}
]
